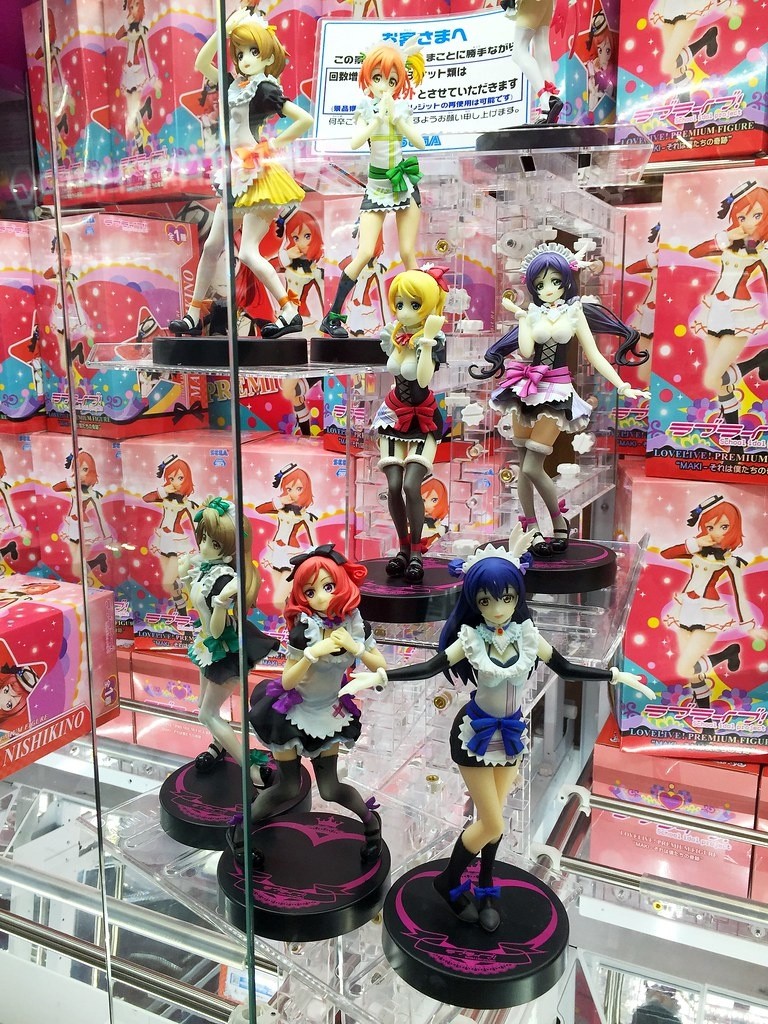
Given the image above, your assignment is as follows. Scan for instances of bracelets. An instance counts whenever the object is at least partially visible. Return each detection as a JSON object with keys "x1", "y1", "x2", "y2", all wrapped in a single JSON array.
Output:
[
  {"x1": 377, "y1": 666, "x2": 388, "y2": 687},
  {"x1": 418, "y1": 337, "x2": 437, "y2": 347},
  {"x1": 392, "y1": 113, "x2": 402, "y2": 126},
  {"x1": 353, "y1": 642, "x2": 366, "y2": 658},
  {"x1": 179, "y1": 575, "x2": 193, "y2": 583},
  {"x1": 212, "y1": 595, "x2": 233, "y2": 609},
  {"x1": 513, "y1": 310, "x2": 527, "y2": 321},
  {"x1": 609, "y1": 667, "x2": 620, "y2": 686},
  {"x1": 375, "y1": 111, "x2": 386, "y2": 125},
  {"x1": 617, "y1": 382, "x2": 631, "y2": 396},
  {"x1": 303, "y1": 646, "x2": 319, "y2": 665},
  {"x1": 268, "y1": 137, "x2": 280, "y2": 154}
]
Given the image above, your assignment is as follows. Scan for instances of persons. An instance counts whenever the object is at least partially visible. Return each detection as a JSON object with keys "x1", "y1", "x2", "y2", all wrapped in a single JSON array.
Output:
[
  {"x1": 334, "y1": 519, "x2": 656, "y2": 934},
  {"x1": 224, "y1": 542, "x2": 389, "y2": 873},
  {"x1": 467, "y1": 238, "x2": 653, "y2": 560},
  {"x1": 167, "y1": 5, "x2": 319, "y2": 342},
  {"x1": 318, "y1": 32, "x2": 429, "y2": 341},
  {"x1": 498, "y1": 0, "x2": 564, "y2": 127},
  {"x1": 368, "y1": 262, "x2": 453, "y2": 584},
  {"x1": 175, "y1": 496, "x2": 283, "y2": 802}
]
